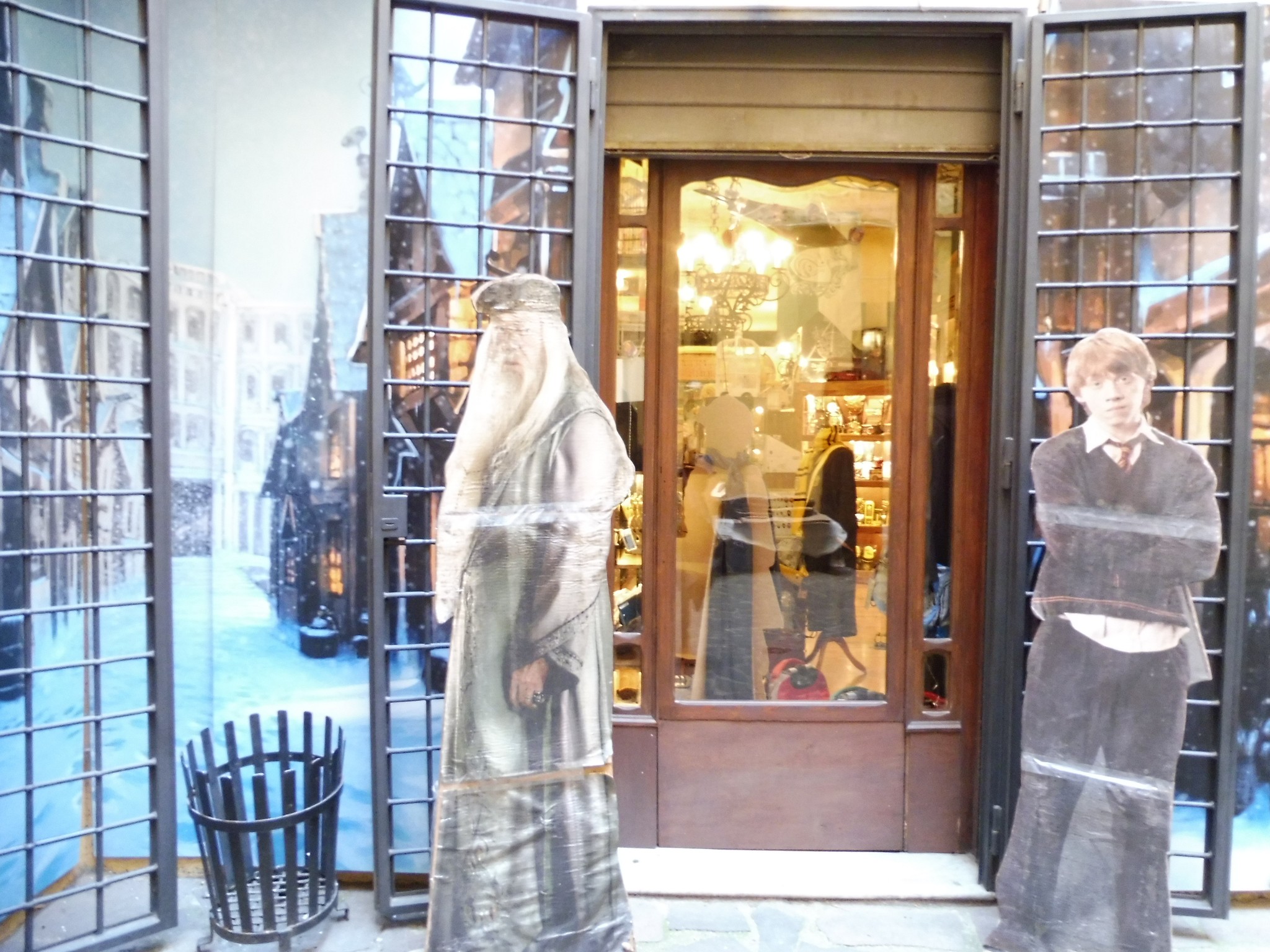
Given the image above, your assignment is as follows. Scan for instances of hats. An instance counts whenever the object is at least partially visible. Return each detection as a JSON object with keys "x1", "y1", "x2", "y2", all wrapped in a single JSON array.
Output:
[{"x1": 471, "y1": 273, "x2": 562, "y2": 314}]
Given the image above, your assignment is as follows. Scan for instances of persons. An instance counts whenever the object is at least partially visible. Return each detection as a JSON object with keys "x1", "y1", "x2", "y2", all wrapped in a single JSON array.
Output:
[
  {"x1": 676, "y1": 391, "x2": 779, "y2": 698},
  {"x1": 927, "y1": 378, "x2": 961, "y2": 710},
  {"x1": 982, "y1": 324, "x2": 1225, "y2": 950},
  {"x1": 799, "y1": 420, "x2": 870, "y2": 681},
  {"x1": 421, "y1": 270, "x2": 647, "y2": 952}
]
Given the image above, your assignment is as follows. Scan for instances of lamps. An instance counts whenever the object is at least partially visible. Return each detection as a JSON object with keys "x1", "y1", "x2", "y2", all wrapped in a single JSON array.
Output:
[{"x1": 676, "y1": 172, "x2": 793, "y2": 346}]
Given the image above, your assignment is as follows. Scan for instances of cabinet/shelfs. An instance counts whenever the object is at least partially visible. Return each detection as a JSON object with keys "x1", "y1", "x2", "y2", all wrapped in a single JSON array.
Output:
[
  {"x1": 795, "y1": 378, "x2": 892, "y2": 584},
  {"x1": 608, "y1": 470, "x2": 645, "y2": 665}
]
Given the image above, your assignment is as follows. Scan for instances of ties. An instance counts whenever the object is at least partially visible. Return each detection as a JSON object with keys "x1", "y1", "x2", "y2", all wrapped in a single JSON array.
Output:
[{"x1": 1106, "y1": 433, "x2": 1147, "y2": 475}]
